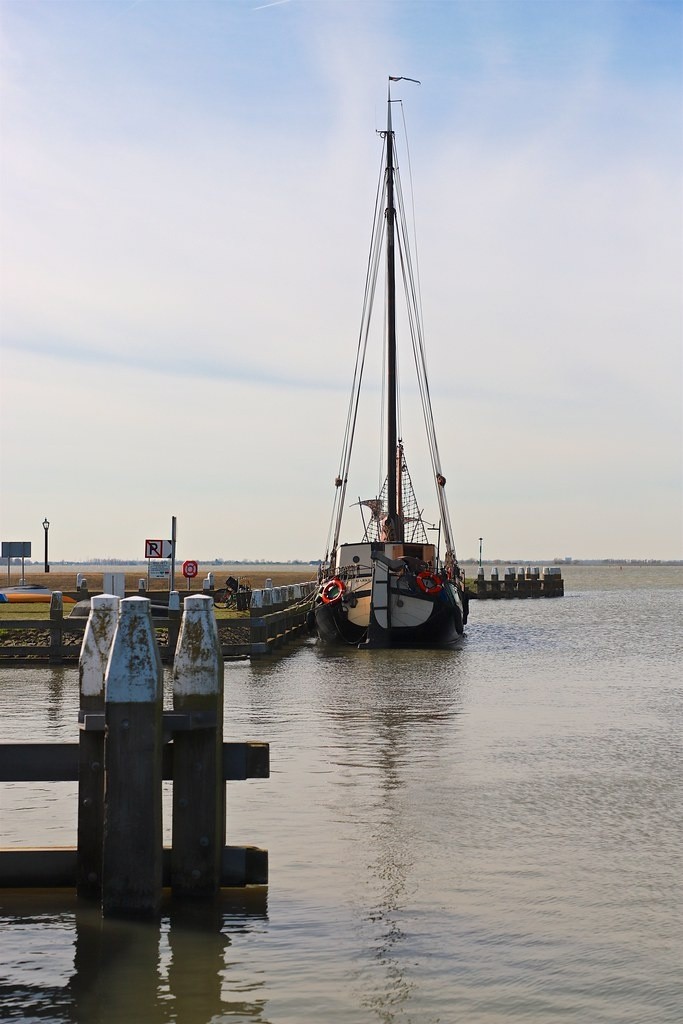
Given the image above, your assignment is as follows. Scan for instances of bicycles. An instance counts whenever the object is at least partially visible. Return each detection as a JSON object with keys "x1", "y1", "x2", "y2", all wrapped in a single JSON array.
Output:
[{"x1": 212, "y1": 576, "x2": 251, "y2": 609}]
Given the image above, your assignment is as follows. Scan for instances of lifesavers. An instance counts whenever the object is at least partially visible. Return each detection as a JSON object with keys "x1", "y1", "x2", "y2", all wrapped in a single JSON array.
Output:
[
  {"x1": 321, "y1": 580, "x2": 344, "y2": 604},
  {"x1": 418, "y1": 571, "x2": 444, "y2": 594}
]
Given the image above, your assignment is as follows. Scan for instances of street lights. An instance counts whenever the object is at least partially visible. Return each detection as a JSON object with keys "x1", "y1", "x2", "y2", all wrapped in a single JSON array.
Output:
[{"x1": 42, "y1": 518, "x2": 50, "y2": 573}]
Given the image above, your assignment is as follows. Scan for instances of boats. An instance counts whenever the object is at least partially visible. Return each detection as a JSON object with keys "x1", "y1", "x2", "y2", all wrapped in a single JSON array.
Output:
[{"x1": 303, "y1": 75, "x2": 470, "y2": 650}]
